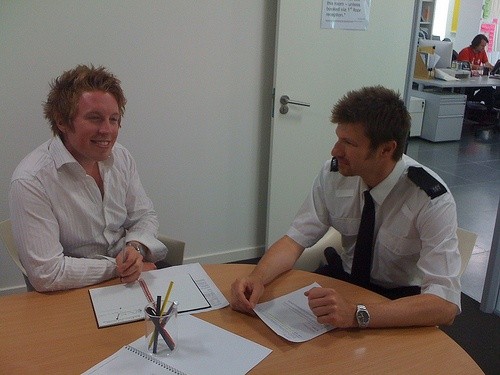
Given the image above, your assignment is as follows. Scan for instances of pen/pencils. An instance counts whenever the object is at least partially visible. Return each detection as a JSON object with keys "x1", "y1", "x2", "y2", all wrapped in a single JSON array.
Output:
[
  {"x1": 120, "y1": 236, "x2": 128, "y2": 285},
  {"x1": 140, "y1": 278, "x2": 179, "y2": 355}
]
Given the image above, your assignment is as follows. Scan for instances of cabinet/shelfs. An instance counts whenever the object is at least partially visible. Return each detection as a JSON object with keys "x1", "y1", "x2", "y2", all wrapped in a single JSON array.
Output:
[
  {"x1": 416, "y1": 0, "x2": 437, "y2": 49},
  {"x1": 413, "y1": 90, "x2": 467, "y2": 142}
]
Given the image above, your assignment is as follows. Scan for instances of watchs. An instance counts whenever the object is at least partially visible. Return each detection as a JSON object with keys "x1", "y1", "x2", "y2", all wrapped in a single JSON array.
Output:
[
  {"x1": 126, "y1": 242, "x2": 149, "y2": 261},
  {"x1": 356, "y1": 303, "x2": 370, "y2": 329}
]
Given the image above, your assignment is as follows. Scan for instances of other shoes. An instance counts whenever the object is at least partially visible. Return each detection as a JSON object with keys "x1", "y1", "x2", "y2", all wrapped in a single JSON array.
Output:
[
  {"x1": 464, "y1": 110, "x2": 480, "y2": 124},
  {"x1": 485, "y1": 109, "x2": 500, "y2": 117}
]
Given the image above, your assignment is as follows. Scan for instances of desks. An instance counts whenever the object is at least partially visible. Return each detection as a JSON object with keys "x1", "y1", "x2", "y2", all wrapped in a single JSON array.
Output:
[
  {"x1": 414, "y1": 75, "x2": 500, "y2": 90},
  {"x1": 0, "y1": 263, "x2": 486, "y2": 375}
]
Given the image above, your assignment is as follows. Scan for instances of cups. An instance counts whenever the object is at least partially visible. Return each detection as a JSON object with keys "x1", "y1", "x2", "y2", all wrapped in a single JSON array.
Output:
[
  {"x1": 483, "y1": 67, "x2": 489, "y2": 76},
  {"x1": 144, "y1": 300, "x2": 177, "y2": 358}
]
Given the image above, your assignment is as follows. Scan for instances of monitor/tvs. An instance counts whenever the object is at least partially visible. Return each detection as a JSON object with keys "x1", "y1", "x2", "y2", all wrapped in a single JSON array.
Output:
[{"x1": 419, "y1": 39, "x2": 453, "y2": 68}]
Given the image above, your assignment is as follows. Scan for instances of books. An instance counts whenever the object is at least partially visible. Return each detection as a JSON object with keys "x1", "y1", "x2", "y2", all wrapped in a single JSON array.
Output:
[{"x1": 88, "y1": 271, "x2": 210, "y2": 328}]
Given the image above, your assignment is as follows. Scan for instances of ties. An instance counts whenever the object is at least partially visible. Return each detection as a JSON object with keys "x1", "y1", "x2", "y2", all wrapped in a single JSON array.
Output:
[{"x1": 349, "y1": 190, "x2": 376, "y2": 289}]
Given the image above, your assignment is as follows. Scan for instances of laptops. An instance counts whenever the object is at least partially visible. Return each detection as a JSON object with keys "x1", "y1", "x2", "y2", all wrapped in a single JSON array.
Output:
[{"x1": 490, "y1": 59, "x2": 500, "y2": 75}]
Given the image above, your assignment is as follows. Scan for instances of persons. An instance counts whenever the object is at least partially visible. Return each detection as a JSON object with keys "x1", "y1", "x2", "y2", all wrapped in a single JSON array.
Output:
[
  {"x1": 9, "y1": 64, "x2": 174, "y2": 293},
  {"x1": 441, "y1": 34, "x2": 496, "y2": 122},
  {"x1": 229, "y1": 85, "x2": 461, "y2": 328}
]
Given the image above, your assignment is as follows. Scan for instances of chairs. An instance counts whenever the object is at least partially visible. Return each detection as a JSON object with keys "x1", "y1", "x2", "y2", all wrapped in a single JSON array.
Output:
[
  {"x1": 291, "y1": 225, "x2": 479, "y2": 278},
  {"x1": 0, "y1": 218, "x2": 186, "y2": 291}
]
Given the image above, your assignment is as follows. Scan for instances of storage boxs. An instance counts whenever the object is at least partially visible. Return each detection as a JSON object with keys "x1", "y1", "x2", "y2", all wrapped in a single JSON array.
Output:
[{"x1": 410, "y1": 96, "x2": 424, "y2": 136}]
